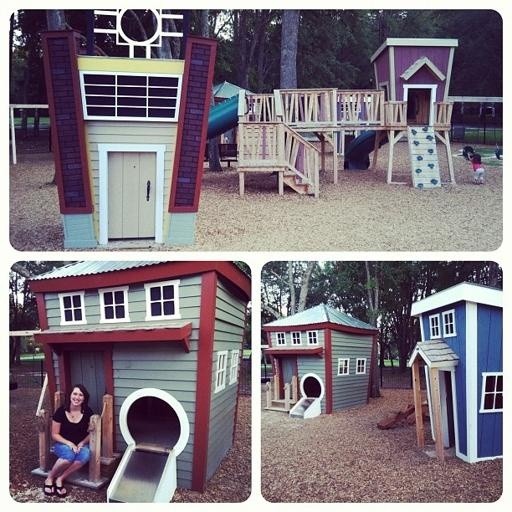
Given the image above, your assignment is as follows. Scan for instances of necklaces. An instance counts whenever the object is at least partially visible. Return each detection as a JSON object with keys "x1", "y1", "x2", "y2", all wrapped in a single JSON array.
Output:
[{"x1": 70, "y1": 412, "x2": 75, "y2": 418}]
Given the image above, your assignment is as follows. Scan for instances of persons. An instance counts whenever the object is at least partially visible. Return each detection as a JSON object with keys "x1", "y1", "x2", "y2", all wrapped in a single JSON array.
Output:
[
  {"x1": 44, "y1": 384, "x2": 94, "y2": 499},
  {"x1": 471, "y1": 154, "x2": 486, "y2": 184}
]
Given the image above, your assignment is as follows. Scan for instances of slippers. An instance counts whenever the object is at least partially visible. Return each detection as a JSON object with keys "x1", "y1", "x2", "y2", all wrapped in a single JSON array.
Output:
[
  {"x1": 44, "y1": 483, "x2": 54, "y2": 496},
  {"x1": 55, "y1": 483, "x2": 68, "y2": 498}
]
